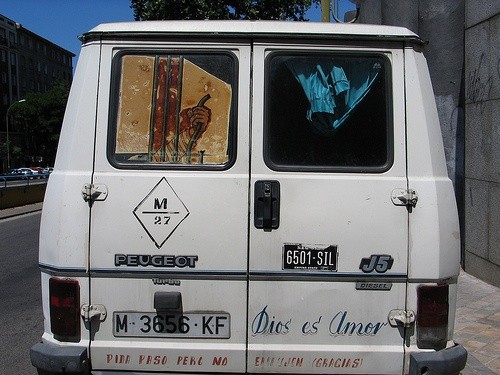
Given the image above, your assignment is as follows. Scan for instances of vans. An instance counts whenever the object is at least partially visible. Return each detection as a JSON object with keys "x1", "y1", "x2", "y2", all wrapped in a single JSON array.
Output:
[{"x1": 28, "y1": 20, "x2": 470, "y2": 375}]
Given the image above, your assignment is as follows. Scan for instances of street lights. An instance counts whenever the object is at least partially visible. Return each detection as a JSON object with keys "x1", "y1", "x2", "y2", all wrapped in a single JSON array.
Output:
[{"x1": 6, "y1": 98, "x2": 26, "y2": 171}]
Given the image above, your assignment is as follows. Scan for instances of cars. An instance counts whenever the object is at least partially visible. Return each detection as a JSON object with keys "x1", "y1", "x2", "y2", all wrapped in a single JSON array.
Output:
[{"x1": 0, "y1": 166, "x2": 54, "y2": 182}]
